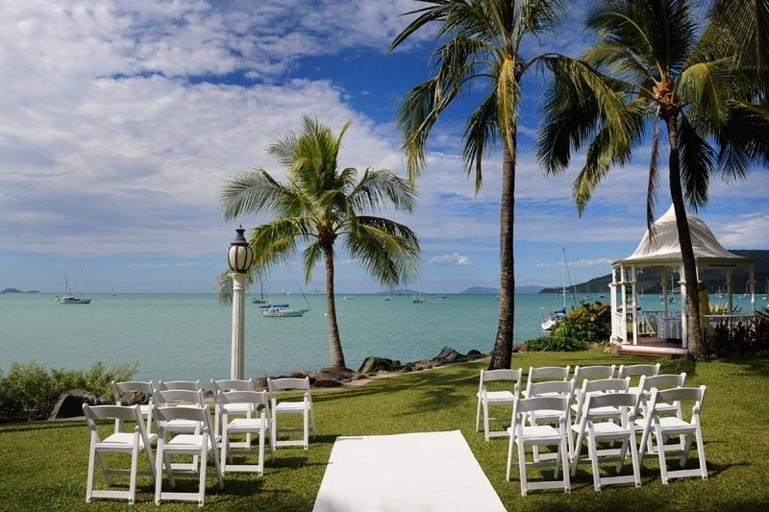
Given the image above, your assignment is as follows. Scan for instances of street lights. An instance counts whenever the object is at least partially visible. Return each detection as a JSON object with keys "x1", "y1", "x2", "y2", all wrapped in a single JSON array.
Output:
[{"x1": 228, "y1": 225, "x2": 253, "y2": 393}]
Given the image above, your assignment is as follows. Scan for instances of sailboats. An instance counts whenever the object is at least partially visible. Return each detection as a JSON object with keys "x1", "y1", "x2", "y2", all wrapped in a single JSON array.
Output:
[
  {"x1": 539, "y1": 247, "x2": 578, "y2": 331},
  {"x1": 582, "y1": 274, "x2": 767, "y2": 304},
  {"x1": 253, "y1": 241, "x2": 312, "y2": 317},
  {"x1": 53, "y1": 268, "x2": 93, "y2": 305}
]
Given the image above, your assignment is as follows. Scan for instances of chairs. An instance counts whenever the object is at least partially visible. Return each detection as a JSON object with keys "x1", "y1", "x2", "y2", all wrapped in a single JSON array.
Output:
[
  {"x1": 81, "y1": 375, "x2": 319, "y2": 508},
  {"x1": 474, "y1": 363, "x2": 710, "y2": 495}
]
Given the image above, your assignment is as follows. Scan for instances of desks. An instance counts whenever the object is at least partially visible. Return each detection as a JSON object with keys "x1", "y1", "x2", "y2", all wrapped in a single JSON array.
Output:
[{"x1": 658, "y1": 316, "x2": 682, "y2": 341}]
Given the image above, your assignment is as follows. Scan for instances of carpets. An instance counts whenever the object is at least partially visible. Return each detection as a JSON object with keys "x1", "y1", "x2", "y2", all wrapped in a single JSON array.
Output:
[{"x1": 310, "y1": 426, "x2": 509, "y2": 511}]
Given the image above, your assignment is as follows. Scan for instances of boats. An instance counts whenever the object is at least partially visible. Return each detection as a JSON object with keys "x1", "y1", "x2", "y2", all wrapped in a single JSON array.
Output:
[{"x1": 343, "y1": 273, "x2": 447, "y2": 304}]
